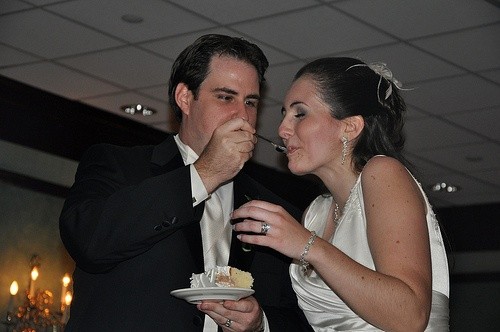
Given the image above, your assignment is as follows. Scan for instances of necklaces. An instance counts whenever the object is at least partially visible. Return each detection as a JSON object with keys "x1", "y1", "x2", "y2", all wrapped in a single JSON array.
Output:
[{"x1": 332, "y1": 196, "x2": 348, "y2": 226}]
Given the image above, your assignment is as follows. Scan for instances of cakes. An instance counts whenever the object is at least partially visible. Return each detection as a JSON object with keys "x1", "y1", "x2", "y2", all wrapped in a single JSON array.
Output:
[{"x1": 190, "y1": 266, "x2": 253, "y2": 289}]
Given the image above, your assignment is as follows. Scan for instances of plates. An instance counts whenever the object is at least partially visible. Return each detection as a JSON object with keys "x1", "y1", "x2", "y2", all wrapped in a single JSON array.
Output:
[{"x1": 170, "y1": 287, "x2": 255, "y2": 304}]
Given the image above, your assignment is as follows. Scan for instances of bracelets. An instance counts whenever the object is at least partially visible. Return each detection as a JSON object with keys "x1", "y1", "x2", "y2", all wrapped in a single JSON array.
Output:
[{"x1": 299, "y1": 231, "x2": 317, "y2": 271}]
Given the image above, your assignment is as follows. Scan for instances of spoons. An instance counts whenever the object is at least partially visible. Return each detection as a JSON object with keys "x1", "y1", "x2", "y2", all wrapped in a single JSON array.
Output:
[{"x1": 254, "y1": 133, "x2": 290, "y2": 153}]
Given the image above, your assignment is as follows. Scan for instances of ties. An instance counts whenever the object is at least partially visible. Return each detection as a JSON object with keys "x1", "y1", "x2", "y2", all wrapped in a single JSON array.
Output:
[{"x1": 200, "y1": 192, "x2": 229, "y2": 332}]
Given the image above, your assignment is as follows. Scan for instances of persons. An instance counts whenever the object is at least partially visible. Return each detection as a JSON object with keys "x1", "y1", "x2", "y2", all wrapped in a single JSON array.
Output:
[
  {"x1": 59, "y1": 34, "x2": 314, "y2": 332},
  {"x1": 229, "y1": 56, "x2": 450, "y2": 332}
]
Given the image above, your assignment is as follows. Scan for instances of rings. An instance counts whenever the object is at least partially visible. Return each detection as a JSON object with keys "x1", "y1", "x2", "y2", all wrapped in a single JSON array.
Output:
[
  {"x1": 223, "y1": 318, "x2": 231, "y2": 327},
  {"x1": 261, "y1": 223, "x2": 270, "y2": 235}
]
